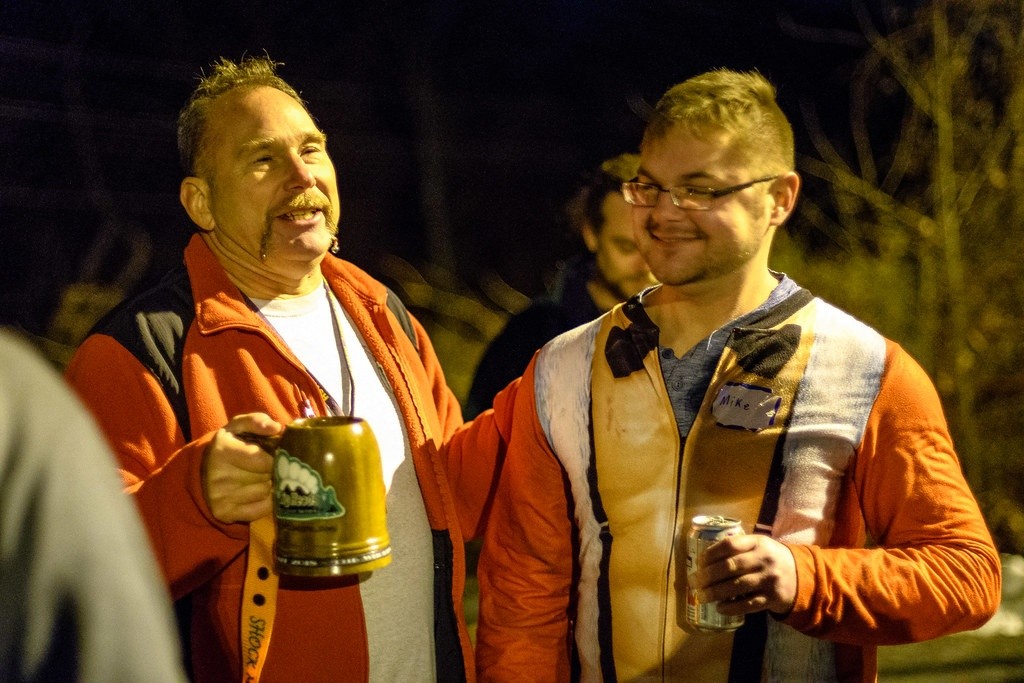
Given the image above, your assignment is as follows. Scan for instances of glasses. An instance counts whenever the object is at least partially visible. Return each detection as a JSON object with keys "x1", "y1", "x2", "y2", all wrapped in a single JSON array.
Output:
[{"x1": 621, "y1": 176, "x2": 780, "y2": 210}]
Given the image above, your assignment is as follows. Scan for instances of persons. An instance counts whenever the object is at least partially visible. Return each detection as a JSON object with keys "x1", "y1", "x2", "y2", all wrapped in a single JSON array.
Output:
[
  {"x1": 473, "y1": 70, "x2": 1000, "y2": 683},
  {"x1": 0, "y1": 332, "x2": 188, "y2": 683},
  {"x1": 461, "y1": 156, "x2": 659, "y2": 425},
  {"x1": 58, "y1": 61, "x2": 522, "y2": 683}
]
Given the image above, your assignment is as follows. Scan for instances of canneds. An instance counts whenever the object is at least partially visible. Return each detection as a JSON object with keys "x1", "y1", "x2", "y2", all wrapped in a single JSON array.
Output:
[{"x1": 686, "y1": 514, "x2": 745, "y2": 633}]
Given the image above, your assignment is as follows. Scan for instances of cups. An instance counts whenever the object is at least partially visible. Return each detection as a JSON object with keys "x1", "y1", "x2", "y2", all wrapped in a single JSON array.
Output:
[{"x1": 242, "y1": 413, "x2": 391, "y2": 578}]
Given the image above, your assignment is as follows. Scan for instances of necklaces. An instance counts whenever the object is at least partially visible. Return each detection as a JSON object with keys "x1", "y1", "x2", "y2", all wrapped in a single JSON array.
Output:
[{"x1": 238, "y1": 279, "x2": 356, "y2": 418}]
